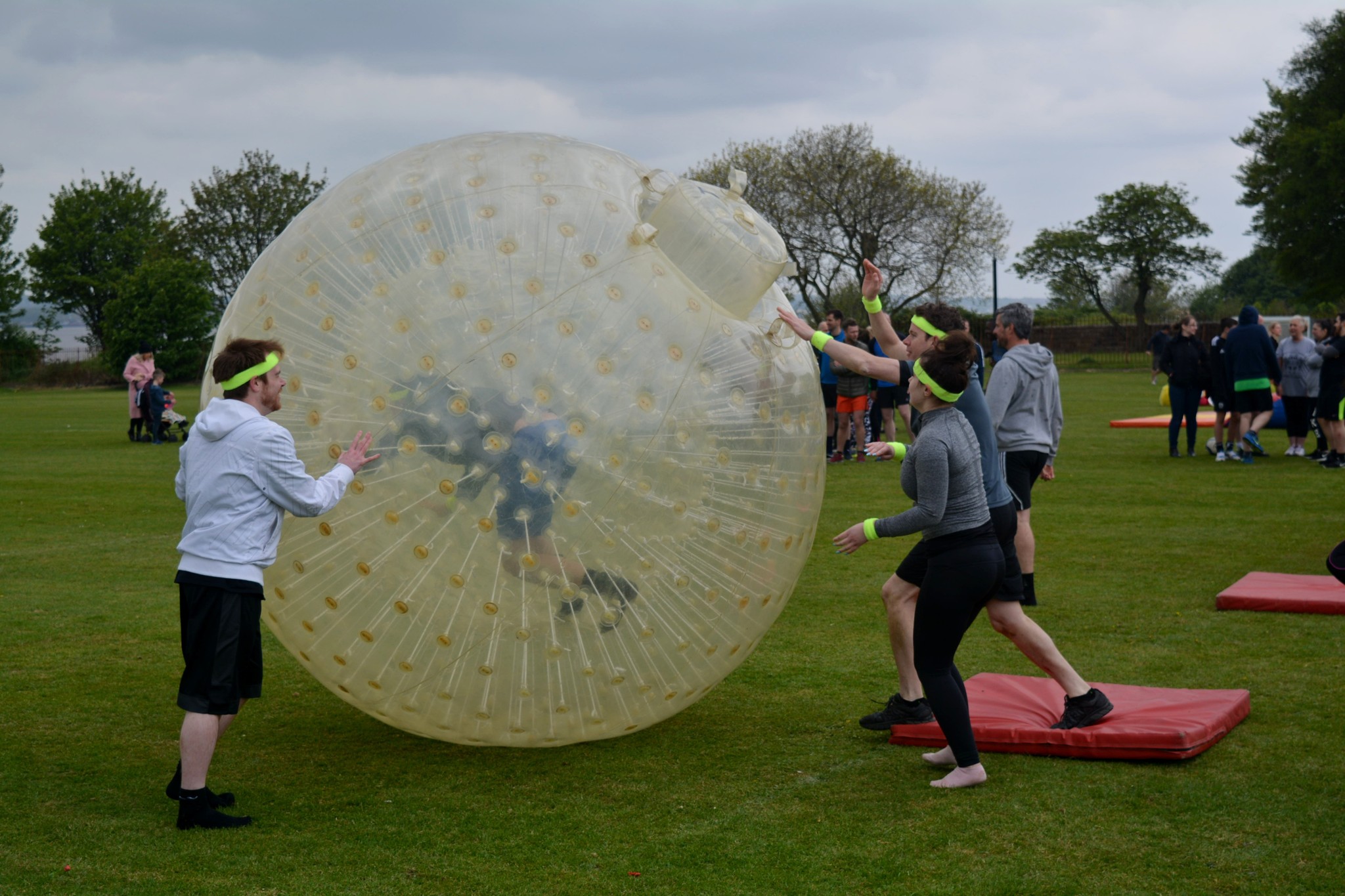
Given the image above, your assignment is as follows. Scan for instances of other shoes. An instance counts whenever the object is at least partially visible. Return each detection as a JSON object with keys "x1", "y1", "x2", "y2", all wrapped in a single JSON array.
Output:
[
  {"x1": 1305, "y1": 449, "x2": 1322, "y2": 459},
  {"x1": 128, "y1": 430, "x2": 135, "y2": 441},
  {"x1": 1187, "y1": 450, "x2": 1195, "y2": 457},
  {"x1": 1284, "y1": 447, "x2": 1294, "y2": 456},
  {"x1": 1226, "y1": 451, "x2": 1241, "y2": 461},
  {"x1": 1319, "y1": 453, "x2": 1345, "y2": 469},
  {"x1": 136, "y1": 432, "x2": 141, "y2": 442},
  {"x1": 1215, "y1": 452, "x2": 1225, "y2": 462},
  {"x1": 1308, "y1": 451, "x2": 1330, "y2": 462},
  {"x1": 1170, "y1": 450, "x2": 1179, "y2": 457},
  {"x1": 875, "y1": 456, "x2": 883, "y2": 463},
  {"x1": 153, "y1": 441, "x2": 163, "y2": 445},
  {"x1": 826, "y1": 454, "x2": 833, "y2": 460},
  {"x1": 1295, "y1": 447, "x2": 1305, "y2": 456}
]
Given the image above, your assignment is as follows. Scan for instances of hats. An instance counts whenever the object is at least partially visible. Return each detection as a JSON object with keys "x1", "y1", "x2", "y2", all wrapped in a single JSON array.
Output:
[{"x1": 138, "y1": 343, "x2": 151, "y2": 354}]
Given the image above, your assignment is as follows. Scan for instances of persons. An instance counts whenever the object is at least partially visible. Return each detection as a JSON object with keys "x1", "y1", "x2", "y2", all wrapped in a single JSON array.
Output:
[
  {"x1": 1326, "y1": 540, "x2": 1345, "y2": 585},
  {"x1": 166, "y1": 336, "x2": 382, "y2": 831},
  {"x1": 774, "y1": 257, "x2": 1114, "y2": 789},
  {"x1": 122, "y1": 342, "x2": 175, "y2": 442},
  {"x1": 361, "y1": 371, "x2": 640, "y2": 633},
  {"x1": 1146, "y1": 304, "x2": 1345, "y2": 469}
]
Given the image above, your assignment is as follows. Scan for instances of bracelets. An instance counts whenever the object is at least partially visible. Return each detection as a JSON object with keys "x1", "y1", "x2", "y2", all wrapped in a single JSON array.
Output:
[
  {"x1": 887, "y1": 441, "x2": 906, "y2": 463},
  {"x1": 863, "y1": 518, "x2": 879, "y2": 540},
  {"x1": 811, "y1": 331, "x2": 834, "y2": 352},
  {"x1": 862, "y1": 295, "x2": 882, "y2": 314}
]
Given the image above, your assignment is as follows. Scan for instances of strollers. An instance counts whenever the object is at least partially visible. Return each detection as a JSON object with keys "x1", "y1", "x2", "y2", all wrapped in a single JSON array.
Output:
[{"x1": 133, "y1": 381, "x2": 189, "y2": 443}]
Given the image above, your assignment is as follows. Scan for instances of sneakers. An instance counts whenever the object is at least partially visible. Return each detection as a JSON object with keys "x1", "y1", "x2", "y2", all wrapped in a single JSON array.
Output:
[
  {"x1": 1242, "y1": 432, "x2": 1264, "y2": 454},
  {"x1": 857, "y1": 453, "x2": 866, "y2": 464},
  {"x1": 1049, "y1": 688, "x2": 1114, "y2": 730},
  {"x1": 828, "y1": 454, "x2": 844, "y2": 464},
  {"x1": 858, "y1": 693, "x2": 936, "y2": 731}
]
